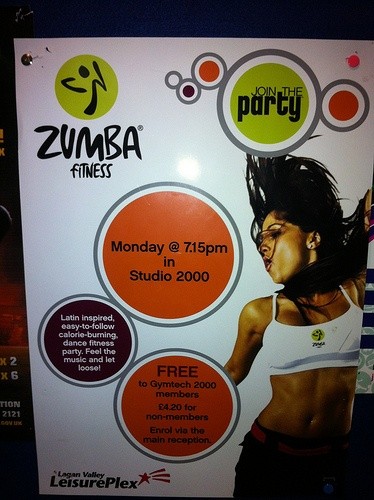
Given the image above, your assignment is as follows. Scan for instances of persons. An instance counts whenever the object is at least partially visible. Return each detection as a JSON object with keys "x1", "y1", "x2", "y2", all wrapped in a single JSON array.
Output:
[{"x1": 222, "y1": 135, "x2": 374, "y2": 500}]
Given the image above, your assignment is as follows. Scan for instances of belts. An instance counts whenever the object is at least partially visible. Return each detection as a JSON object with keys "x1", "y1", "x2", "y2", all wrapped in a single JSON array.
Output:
[{"x1": 249, "y1": 423, "x2": 349, "y2": 456}]
